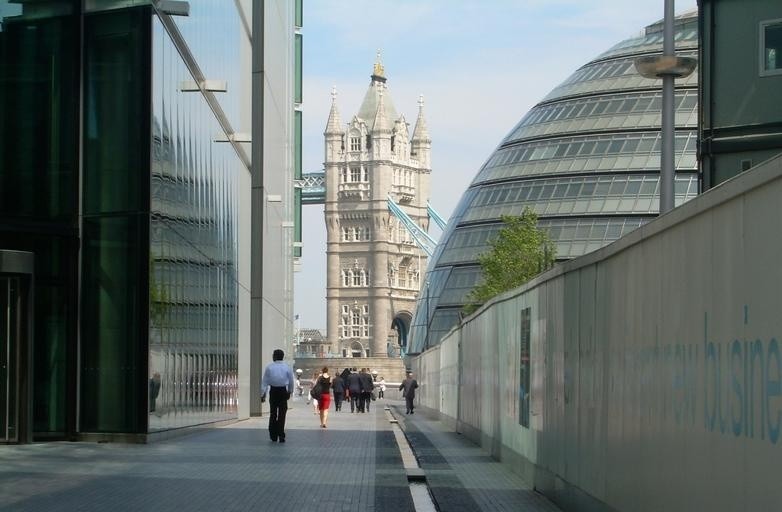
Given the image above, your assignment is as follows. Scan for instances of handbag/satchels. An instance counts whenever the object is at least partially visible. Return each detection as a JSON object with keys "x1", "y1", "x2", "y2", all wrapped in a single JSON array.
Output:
[{"x1": 310, "y1": 383, "x2": 321, "y2": 401}]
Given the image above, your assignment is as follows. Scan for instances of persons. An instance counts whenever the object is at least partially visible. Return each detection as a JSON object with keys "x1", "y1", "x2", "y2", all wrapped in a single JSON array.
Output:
[
  {"x1": 260, "y1": 349, "x2": 295, "y2": 443},
  {"x1": 307, "y1": 365, "x2": 386, "y2": 415},
  {"x1": 399, "y1": 373, "x2": 420, "y2": 414},
  {"x1": 314, "y1": 366, "x2": 332, "y2": 429}
]
